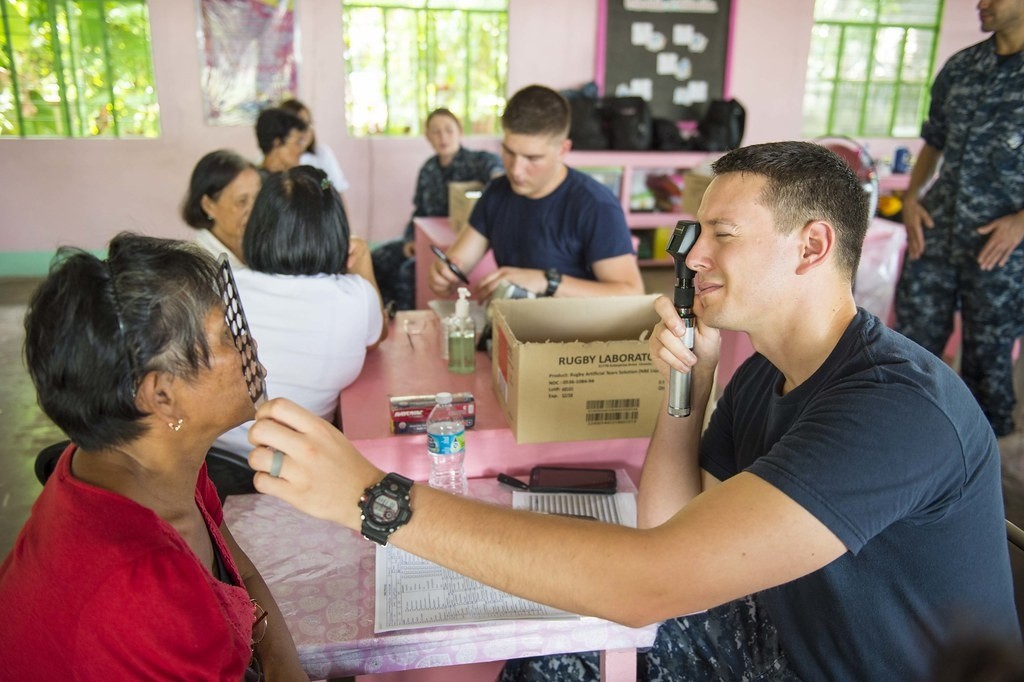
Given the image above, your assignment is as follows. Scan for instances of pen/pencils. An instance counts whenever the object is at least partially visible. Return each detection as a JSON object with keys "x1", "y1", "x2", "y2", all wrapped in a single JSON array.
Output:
[{"x1": 530, "y1": 510, "x2": 597, "y2": 521}]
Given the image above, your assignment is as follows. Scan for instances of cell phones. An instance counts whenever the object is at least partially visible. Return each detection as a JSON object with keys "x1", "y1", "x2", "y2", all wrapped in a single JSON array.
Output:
[{"x1": 529, "y1": 465, "x2": 617, "y2": 495}]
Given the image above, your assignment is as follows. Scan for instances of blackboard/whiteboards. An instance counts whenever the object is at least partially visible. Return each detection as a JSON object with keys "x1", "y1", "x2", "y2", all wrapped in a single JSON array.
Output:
[{"x1": 596, "y1": 0, "x2": 737, "y2": 129}]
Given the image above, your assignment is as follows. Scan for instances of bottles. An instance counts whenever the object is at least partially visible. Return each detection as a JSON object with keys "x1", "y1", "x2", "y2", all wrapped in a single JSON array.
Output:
[{"x1": 426, "y1": 391, "x2": 468, "y2": 496}]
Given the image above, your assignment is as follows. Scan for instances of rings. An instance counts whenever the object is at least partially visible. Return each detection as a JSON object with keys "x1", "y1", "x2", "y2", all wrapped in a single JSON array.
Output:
[{"x1": 271, "y1": 452, "x2": 284, "y2": 476}]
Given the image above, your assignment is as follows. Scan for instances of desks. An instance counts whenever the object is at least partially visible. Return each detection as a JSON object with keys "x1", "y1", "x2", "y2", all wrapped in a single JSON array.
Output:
[{"x1": 223, "y1": 219, "x2": 907, "y2": 682}]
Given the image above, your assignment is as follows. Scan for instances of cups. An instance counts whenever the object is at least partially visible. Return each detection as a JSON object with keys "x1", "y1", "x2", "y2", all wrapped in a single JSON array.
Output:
[{"x1": 892, "y1": 147, "x2": 909, "y2": 172}]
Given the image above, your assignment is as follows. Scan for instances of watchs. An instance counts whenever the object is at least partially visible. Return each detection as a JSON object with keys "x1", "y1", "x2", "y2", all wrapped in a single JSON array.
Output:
[
  {"x1": 545, "y1": 268, "x2": 561, "y2": 296},
  {"x1": 359, "y1": 472, "x2": 415, "y2": 545}
]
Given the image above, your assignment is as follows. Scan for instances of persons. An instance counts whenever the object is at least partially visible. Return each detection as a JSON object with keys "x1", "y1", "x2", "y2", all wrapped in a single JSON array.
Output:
[
  {"x1": 897, "y1": 0, "x2": 1024, "y2": 438},
  {"x1": 428, "y1": 86, "x2": 647, "y2": 307},
  {"x1": 282, "y1": 99, "x2": 347, "y2": 194},
  {"x1": 247, "y1": 140, "x2": 1023, "y2": 682},
  {"x1": 372, "y1": 109, "x2": 504, "y2": 311},
  {"x1": 210, "y1": 164, "x2": 387, "y2": 461},
  {"x1": 182, "y1": 150, "x2": 262, "y2": 270},
  {"x1": 0, "y1": 232, "x2": 308, "y2": 681},
  {"x1": 256, "y1": 109, "x2": 308, "y2": 178}
]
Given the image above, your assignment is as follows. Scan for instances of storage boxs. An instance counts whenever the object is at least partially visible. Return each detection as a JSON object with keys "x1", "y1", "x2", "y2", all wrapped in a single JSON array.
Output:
[
  {"x1": 449, "y1": 182, "x2": 485, "y2": 234},
  {"x1": 491, "y1": 293, "x2": 717, "y2": 444}
]
